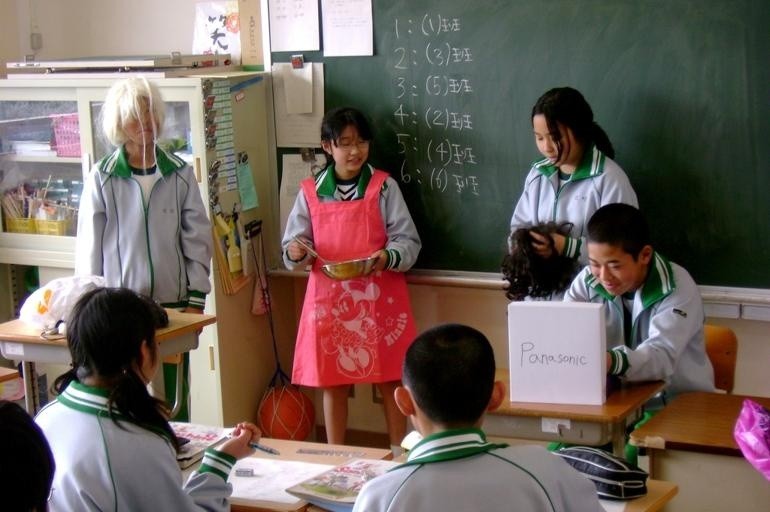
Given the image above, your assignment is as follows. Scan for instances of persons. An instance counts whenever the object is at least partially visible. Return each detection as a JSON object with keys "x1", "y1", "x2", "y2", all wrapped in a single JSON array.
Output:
[
  {"x1": 281, "y1": 108, "x2": 421, "y2": 459},
  {"x1": 508, "y1": 87, "x2": 638, "y2": 302},
  {"x1": 565, "y1": 203, "x2": 715, "y2": 464},
  {"x1": 350, "y1": 324, "x2": 607, "y2": 512},
  {"x1": 33, "y1": 286, "x2": 262, "y2": 512},
  {"x1": 76, "y1": 76, "x2": 213, "y2": 423},
  {"x1": 1, "y1": 399, "x2": 56, "y2": 512}
]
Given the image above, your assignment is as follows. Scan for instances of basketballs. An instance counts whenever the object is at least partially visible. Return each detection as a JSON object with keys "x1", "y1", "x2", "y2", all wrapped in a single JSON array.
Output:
[{"x1": 258, "y1": 383, "x2": 315, "y2": 440}]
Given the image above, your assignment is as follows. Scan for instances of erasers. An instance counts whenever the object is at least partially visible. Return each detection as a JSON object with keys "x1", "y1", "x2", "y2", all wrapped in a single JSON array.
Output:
[{"x1": 236, "y1": 468, "x2": 254, "y2": 477}]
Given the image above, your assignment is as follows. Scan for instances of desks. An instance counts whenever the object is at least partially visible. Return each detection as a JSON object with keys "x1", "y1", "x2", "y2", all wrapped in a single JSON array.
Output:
[{"x1": 0, "y1": 307, "x2": 769, "y2": 510}]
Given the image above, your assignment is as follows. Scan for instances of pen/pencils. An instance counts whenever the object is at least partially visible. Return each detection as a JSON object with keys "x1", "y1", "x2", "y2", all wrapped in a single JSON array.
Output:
[
  {"x1": 5, "y1": 175, "x2": 78, "y2": 221},
  {"x1": 228, "y1": 433, "x2": 280, "y2": 454}
]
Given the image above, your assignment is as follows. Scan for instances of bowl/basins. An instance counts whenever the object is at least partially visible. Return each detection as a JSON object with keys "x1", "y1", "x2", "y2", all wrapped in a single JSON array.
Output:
[{"x1": 319, "y1": 258, "x2": 380, "y2": 280}]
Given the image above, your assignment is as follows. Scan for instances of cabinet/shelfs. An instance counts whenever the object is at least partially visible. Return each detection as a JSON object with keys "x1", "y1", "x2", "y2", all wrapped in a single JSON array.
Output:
[{"x1": 0, "y1": 67, "x2": 319, "y2": 442}]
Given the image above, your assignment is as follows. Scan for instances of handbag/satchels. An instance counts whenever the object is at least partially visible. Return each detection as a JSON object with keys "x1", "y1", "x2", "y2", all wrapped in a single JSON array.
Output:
[
  {"x1": 20, "y1": 273, "x2": 105, "y2": 337},
  {"x1": 249, "y1": 277, "x2": 274, "y2": 315}
]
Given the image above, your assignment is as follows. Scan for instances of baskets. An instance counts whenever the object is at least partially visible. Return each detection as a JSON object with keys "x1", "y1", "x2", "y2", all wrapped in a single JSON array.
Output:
[
  {"x1": 5, "y1": 218, "x2": 71, "y2": 235},
  {"x1": 50, "y1": 113, "x2": 82, "y2": 158}
]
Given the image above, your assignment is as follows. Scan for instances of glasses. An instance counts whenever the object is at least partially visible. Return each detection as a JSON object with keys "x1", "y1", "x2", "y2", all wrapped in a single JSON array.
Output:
[{"x1": 335, "y1": 137, "x2": 367, "y2": 148}]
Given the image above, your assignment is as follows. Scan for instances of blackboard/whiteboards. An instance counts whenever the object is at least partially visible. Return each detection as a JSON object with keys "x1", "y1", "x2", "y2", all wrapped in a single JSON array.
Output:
[{"x1": 260, "y1": 1, "x2": 769, "y2": 307}]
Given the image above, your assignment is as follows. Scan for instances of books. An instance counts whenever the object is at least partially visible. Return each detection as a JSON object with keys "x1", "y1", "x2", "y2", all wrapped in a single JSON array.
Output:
[
  {"x1": 226, "y1": 456, "x2": 404, "y2": 512},
  {"x1": 6, "y1": 127, "x2": 59, "y2": 157}
]
